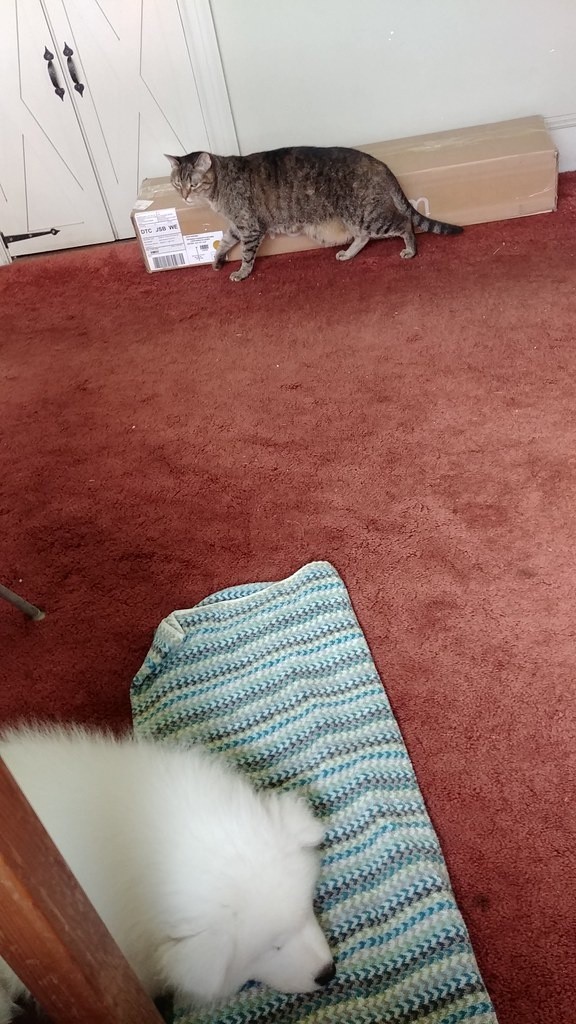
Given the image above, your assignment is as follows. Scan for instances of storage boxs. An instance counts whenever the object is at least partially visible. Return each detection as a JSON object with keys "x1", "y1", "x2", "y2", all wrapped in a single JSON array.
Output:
[{"x1": 130, "y1": 115, "x2": 561, "y2": 275}]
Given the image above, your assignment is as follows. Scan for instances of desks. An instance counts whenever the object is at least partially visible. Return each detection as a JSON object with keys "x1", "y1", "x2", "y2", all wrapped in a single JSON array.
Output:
[{"x1": 0, "y1": 753, "x2": 164, "y2": 1023}]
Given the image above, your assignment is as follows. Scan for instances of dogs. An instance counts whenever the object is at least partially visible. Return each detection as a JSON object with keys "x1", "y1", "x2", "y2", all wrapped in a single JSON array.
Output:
[{"x1": 0, "y1": 720, "x2": 337, "y2": 1024}]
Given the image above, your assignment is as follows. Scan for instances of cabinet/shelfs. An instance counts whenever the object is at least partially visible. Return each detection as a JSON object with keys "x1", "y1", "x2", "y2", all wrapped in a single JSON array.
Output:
[{"x1": 2, "y1": 0, "x2": 246, "y2": 262}]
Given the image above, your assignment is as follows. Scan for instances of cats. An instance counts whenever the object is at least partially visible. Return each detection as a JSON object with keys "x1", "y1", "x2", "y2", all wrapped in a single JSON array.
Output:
[{"x1": 163, "y1": 146, "x2": 465, "y2": 282}]
